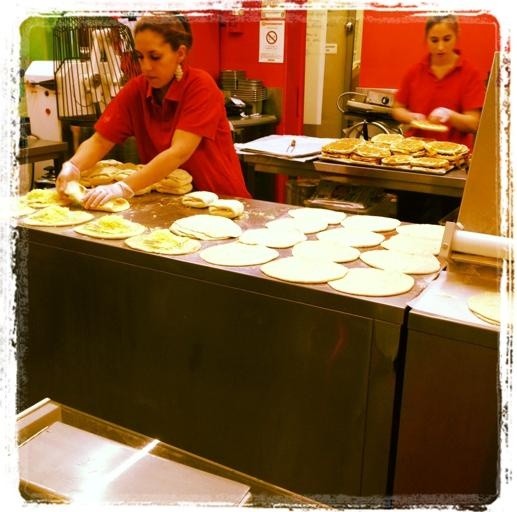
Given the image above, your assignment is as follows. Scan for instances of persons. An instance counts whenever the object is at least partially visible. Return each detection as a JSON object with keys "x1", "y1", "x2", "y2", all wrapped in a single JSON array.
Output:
[
  {"x1": 390, "y1": 10, "x2": 486, "y2": 224},
  {"x1": 52, "y1": 10, "x2": 254, "y2": 212}
]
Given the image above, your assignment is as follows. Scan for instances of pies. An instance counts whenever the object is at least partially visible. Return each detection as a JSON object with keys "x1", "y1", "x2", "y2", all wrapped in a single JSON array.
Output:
[
  {"x1": 23, "y1": 159, "x2": 500, "y2": 326},
  {"x1": 321, "y1": 133, "x2": 470, "y2": 170}
]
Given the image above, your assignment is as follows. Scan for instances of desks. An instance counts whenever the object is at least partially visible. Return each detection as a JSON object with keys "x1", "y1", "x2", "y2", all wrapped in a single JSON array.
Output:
[{"x1": 242, "y1": 155, "x2": 467, "y2": 197}]
